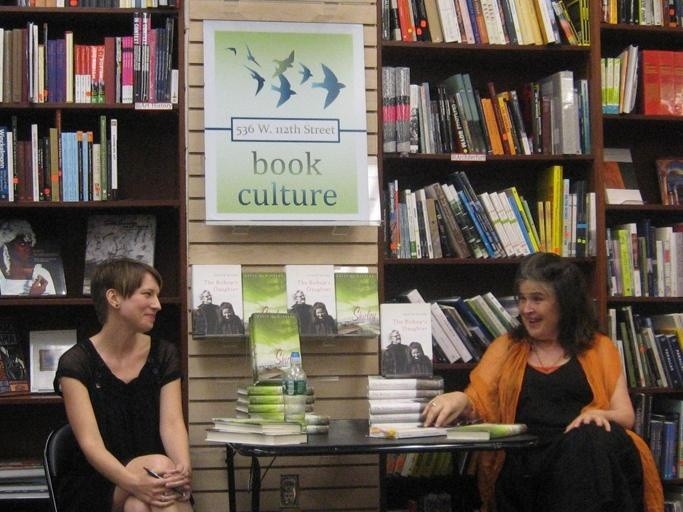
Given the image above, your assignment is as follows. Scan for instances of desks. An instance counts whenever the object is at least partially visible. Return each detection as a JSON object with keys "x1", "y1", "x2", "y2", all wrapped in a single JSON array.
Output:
[{"x1": 226, "y1": 415, "x2": 537, "y2": 511}]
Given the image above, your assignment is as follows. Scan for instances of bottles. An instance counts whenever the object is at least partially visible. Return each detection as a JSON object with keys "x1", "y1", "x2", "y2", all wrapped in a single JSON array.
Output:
[{"x1": 282, "y1": 350, "x2": 307, "y2": 425}]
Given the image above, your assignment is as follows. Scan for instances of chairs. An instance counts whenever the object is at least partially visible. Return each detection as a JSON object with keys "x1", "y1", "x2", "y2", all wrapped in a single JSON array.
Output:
[{"x1": 42, "y1": 417, "x2": 124, "y2": 512}]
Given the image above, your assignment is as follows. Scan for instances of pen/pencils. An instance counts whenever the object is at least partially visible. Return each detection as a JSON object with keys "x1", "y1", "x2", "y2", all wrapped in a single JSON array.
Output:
[{"x1": 143, "y1": 467, "x2": 184, "y2": 497}]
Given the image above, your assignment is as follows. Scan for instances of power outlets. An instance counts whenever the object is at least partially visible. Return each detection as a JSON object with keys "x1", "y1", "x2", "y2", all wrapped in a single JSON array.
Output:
[{"x1": 279, "y1": 471, "x2": 297, "y2": 508}]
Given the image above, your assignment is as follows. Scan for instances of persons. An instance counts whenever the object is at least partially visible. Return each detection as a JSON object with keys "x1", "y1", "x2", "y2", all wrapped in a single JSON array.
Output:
[
  {"x1": 53, "y1": 257, "x2": 193, "y2": 512},
  {"x1": 423, "y1": 250, "x2": 664, "y2": 512},
  {"x1": 601, "y1": 0, "x2": 683, "y2": 481}
]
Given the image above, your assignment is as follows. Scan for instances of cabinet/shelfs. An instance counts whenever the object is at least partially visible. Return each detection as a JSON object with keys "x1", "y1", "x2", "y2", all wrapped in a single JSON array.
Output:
[
  {"x1": 189, "y1": 214, "x2": 380, "y2": 344},
  {"x1": 1, "y1": 1, "x2": 183, "y2": 471},
  {"x1": 592, "y1": 1, "x2": 683, "y2": 493},
  {"x1": 377, "y1": 1, "x2": 602, "y2": 512}
]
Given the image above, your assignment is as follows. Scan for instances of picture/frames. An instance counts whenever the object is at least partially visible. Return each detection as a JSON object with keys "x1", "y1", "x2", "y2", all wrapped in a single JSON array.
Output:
[{"x1": 203, "y1": 19, "x2": 371, "y2": 227}]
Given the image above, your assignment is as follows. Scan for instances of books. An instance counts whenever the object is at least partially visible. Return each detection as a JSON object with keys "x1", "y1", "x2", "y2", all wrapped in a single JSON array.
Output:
[
  {"x1": 29, "y1": 329, "x2": 77, "y2": 394},
  {"x1": 0, "y1": 212, "x2": 67, "y2": 295},
  {"x1": 0, "y1": 12, "x2": 179, "y2": 104},
  {"x1": 192, "y1": 264, "x2": 381, "y2": 448},
  {"x1": 83, "y1": 212, "x2": 156, "y2": 294},
  {"x1": 380, "y1": 0, "x2": 596, "y2": 360},
  {"x1": 0, "y1": 109, "x2": 118, "y2": 202},
  {"x1": 368, "y1": 303, "x2": 447, "y2": 439},
  {"x1": 0, "y1": 453, "x2": 50, "y2": 499},
  {"x1": 386, "y1": 451, "x2": 477, "y2": 476},
  {"x1": 0, "y1": 316, "x2": 30, "y2": 393},
  {"x1": 18, "y1": 0, "x2": 181, "y2": 9}
]
